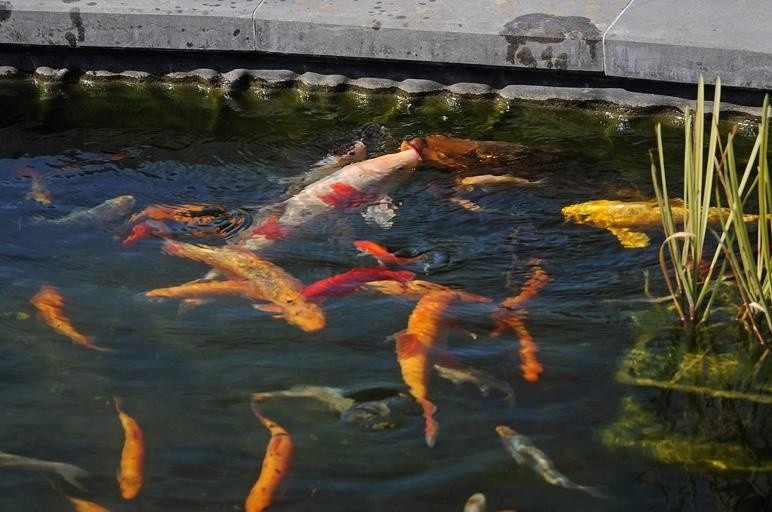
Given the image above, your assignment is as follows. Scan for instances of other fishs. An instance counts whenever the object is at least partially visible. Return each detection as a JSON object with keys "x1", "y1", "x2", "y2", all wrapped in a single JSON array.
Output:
[{"x1": 1, "y1": 131, "x2": 769, "y2": 512}]
